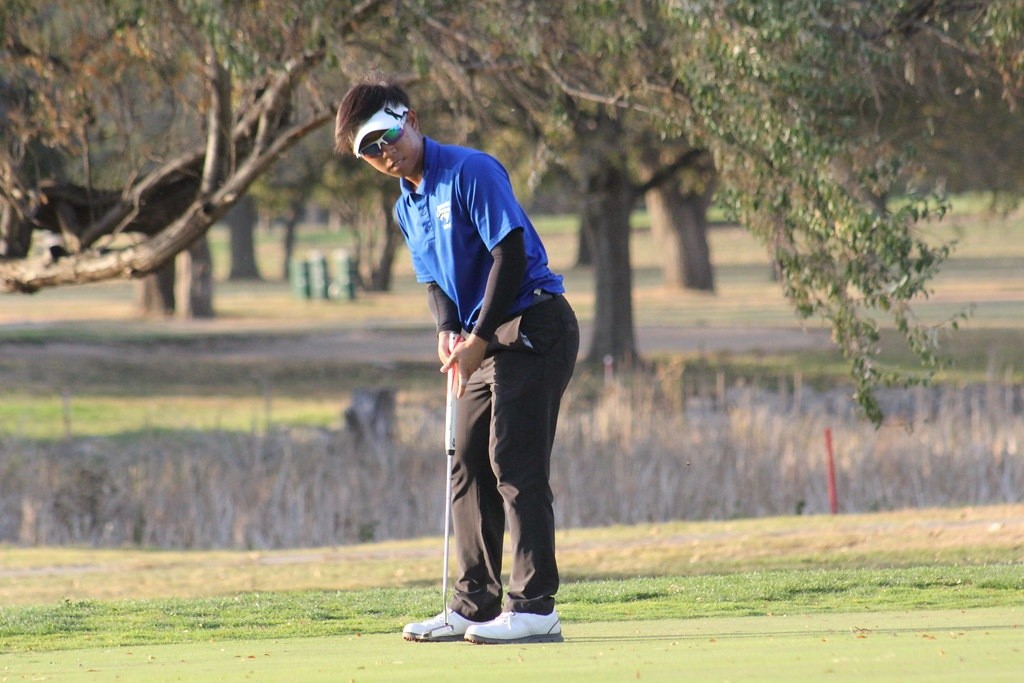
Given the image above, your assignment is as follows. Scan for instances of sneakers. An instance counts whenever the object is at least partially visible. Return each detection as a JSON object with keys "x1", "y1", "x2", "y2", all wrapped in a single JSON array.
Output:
[
  {"x1": 402, "y1": 606, "x2": 496, "y2": 642},
  {"x1": 464, "y1": 602, "x2": 566, "y2": 644}
]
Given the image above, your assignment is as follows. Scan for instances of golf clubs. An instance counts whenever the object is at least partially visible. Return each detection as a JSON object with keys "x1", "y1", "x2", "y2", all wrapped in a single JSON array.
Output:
[{"x1": 422, "y1": 327, "x2": 460, "y2": 638}]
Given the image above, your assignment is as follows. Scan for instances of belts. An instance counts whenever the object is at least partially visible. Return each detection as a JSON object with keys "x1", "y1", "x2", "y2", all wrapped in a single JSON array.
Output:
[{"x1": 526, "y1": 288, "x2": 564, "y2": 306}]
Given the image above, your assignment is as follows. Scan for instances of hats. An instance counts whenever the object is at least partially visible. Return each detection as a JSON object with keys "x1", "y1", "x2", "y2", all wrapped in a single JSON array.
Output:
[{"x1": 350, "y1": 100, "x2": 411, "y2": 161}]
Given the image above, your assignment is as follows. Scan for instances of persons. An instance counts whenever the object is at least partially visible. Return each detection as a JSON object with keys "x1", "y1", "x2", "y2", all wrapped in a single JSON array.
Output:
[{"x1": 334, "y1": 69, "x2": 580, "y2": 644}]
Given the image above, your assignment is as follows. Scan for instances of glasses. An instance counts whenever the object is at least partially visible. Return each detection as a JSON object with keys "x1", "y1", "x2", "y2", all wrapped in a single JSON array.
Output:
[{"x1": 359, "y1": 112, "x2": 409, "y2": 159}]
